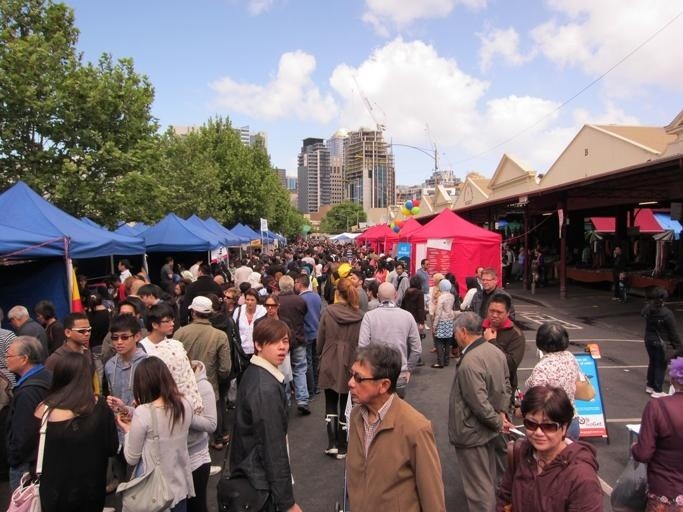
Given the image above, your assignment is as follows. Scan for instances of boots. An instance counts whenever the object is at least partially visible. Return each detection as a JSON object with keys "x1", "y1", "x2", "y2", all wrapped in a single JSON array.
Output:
[
  {"x1": 335, "y1": 420, "x2": 349, "y2": 460},
  {"x1": 324, "y1": 413, "x2": 339, "y2": 455}
]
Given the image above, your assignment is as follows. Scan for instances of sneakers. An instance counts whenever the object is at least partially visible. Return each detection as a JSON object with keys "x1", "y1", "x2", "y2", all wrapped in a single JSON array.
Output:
[
  {"x1": 645, "y1": 385, "x2": 672, "y2": 398},
  {"x1": 298, "y1": 404, "x2": 311, "y2": 415},
  {"x1": 430, "y1": 346, "x2": 459, "y2": 359},
  {"x1": 209, "y1": 433, "x2": 230, "y2": 450},
  {"x1": 209, "y1": 464, "x2": 222, "y2": 476}
]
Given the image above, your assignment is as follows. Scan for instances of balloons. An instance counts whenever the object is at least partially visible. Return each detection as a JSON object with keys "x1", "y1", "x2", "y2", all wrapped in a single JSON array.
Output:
[{"x1": 388, "y1": 198, "x2": 420, "y2": 234}]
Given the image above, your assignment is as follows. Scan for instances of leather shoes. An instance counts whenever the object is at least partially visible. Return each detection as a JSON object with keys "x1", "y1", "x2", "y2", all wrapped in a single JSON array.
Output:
[{"x1": 431, "y1": 363, "x2": 445, "y2": 368}]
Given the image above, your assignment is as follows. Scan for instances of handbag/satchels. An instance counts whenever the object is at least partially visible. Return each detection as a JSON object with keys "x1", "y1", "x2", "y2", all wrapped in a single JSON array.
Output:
[
  {"x1": 216, "y1": 476, "x2": 259, "y2": 511},
  {"x1": 7, "y1": 473, "x2": 42, "y2": 511},
  {"x1": 575, "y1": 367, "x2": 598, "y2": 403},
  {"x1": 114, "y1": 465, "x2": 176, "y2": 512},
  {"x1": 230, "y1": 328, "x2": 247, "y2": 378}
]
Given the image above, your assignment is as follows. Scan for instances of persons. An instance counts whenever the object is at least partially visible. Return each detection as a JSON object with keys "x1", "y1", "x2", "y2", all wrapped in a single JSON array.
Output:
[{"x1": 0, "y1": 237, "x2": 682, "y2": 512}]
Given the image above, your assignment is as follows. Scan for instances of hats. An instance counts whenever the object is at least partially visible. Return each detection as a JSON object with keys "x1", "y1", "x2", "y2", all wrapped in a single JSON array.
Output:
[{"x1": 188, "y1": 296, "x2": 214, "y2": 314}]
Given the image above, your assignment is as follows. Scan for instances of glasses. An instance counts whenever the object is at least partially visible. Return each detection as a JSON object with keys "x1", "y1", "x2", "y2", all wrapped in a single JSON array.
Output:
[
  {"x1": 524, "y1": 419, "x2": 558, "y2": 432},
  {"x1": 71, "y1": 327, "x2": 93, "y2": 334},
  {"x1": 348, "y1": 368, "x2": 381, "y2": 384},
  {"x1": 111, "y1": 335, "x2": 135, "y2": 340}
]
